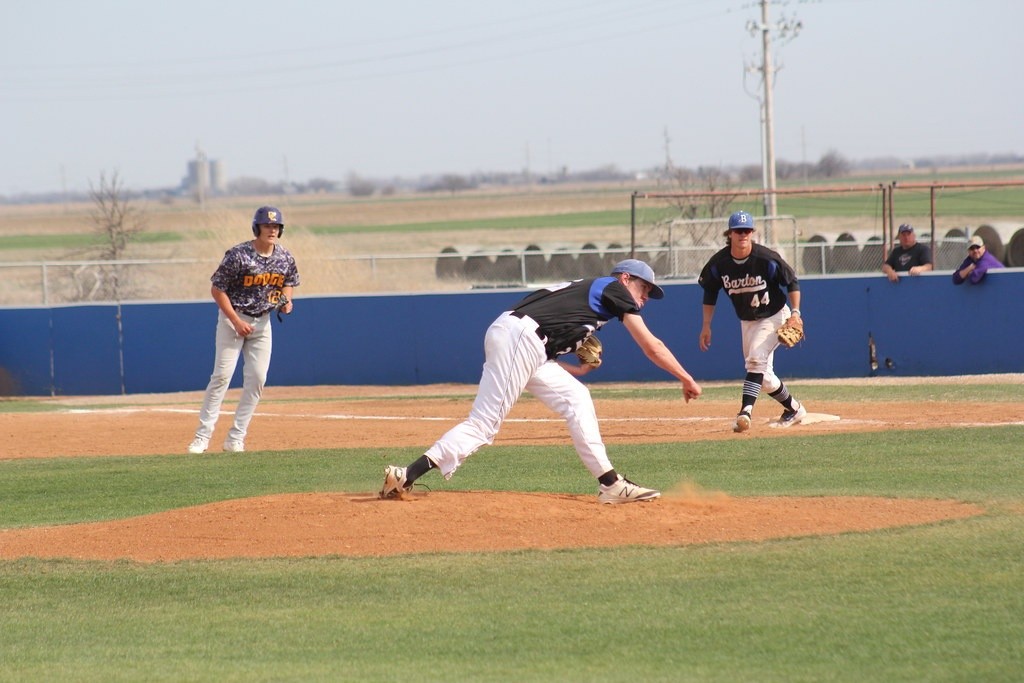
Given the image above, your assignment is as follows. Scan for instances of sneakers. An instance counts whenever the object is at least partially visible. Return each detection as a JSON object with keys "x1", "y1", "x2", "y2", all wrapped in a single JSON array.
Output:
[
  {"x1": 767, "y1": 402, "x2": 806, "y2": 428},
  {"x1": 598, "y1": 474, "x2": 662, "y2": 503},
  {"x1": 380, "y1": 464, "x2": 415, "y2": 499},
  {"x1": 223, "y1": 442, "x2": 244, "y2": 452},
  {"x1": 733, "y1": 410, "x2": 751, "y2": 433},
  {"x1": 185, "y1": 438, "x2": 209, "y2": 453}
]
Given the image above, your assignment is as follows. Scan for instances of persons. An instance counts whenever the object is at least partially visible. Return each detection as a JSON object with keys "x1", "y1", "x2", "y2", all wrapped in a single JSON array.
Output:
[
  {"x1": 882, "y1": 223, "x2": 933, "y2": 284},
  {"x1": 698, "y1": 210, "x2": 807, "y2": 433},
  {"x1": 377, "y1": 258, "x2": 702, "y2": 505},
  {"x1": 952, "y1": 235, "x2": 1006, "y2": 286},
  {"x1": 188, "y1": 206, "x2": 300, "y2": 454}
]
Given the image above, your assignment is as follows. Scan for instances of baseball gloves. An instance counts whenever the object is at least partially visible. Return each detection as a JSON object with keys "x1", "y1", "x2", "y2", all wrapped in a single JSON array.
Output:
[
  {"x1": 774, "y1": 317, "x2": 805, "y2": 348},
  {"x1": 576, "y1": 334, "x2": 604, "y2": 370}
]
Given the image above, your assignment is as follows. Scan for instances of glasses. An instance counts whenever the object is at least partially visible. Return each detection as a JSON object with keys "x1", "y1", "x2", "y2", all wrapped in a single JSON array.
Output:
[{"x1": 735, "y1": 229, "x2": 752, "y2": 235}]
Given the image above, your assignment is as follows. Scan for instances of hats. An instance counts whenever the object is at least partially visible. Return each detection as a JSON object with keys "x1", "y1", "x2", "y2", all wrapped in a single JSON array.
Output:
[
  {"x1": 898, "y1": 224, "x2": 914, "y2": 234},
  {"x1": 252, "y1": 206, "x2": 285, "y2": 239},
  {"x1": 610, "y1": 259, "x2": 665, "y2": 299},
  {"x1": 722, "y1": 211, "x2": 755, "y2": 236},
  {"x1": 965, "y1": 236, "x2": 983, "y2": 250}
]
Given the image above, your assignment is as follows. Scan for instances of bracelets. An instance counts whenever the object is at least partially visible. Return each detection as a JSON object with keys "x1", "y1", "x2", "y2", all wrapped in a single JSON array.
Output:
[{"x1": 791, "y1": 309, "x2": 801, "y2": 316}]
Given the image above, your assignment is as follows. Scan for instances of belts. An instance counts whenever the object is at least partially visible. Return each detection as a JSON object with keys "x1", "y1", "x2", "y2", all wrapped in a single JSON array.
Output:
[
  {"x1": 509, "y1": 311, "x2": 552, "y2": 361},
  {"x1": 234, "y1": 306, "x2": 272, "y2": 317}
]
